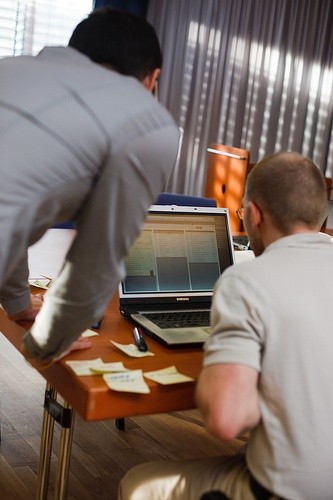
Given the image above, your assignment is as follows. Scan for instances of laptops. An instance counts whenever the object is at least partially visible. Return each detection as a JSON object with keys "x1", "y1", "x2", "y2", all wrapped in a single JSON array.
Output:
[{"x1": 117, "y1": 205, "x2": 233, "y2": 346}]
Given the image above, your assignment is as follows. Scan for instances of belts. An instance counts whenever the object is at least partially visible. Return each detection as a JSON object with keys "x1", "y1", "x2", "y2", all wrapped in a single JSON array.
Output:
[{"x1": 248, "y1": 471, "x2": 275, "y2": 500}]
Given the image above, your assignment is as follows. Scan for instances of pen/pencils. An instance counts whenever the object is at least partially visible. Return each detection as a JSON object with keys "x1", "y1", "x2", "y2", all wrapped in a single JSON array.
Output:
[{"x1": 133, "y1": 327, "x2": 148, "y2": 352}]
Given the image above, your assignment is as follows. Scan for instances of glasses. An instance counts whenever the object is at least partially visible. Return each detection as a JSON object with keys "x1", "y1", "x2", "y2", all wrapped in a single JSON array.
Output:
[{"x1": 235, "y1": 206, "x2": 247, "y2": 220}]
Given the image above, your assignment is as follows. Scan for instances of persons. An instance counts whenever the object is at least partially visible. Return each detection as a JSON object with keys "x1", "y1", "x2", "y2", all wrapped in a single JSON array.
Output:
[
  {"x1": 0, "y1": 5, "x2": 183, "y2": 370},
  {"x1": 119, "y1": 153, "x2": 333, "y2": 500}
]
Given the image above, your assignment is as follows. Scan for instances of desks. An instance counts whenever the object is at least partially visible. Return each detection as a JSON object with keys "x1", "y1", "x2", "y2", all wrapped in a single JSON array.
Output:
[{"x1": 0, "y1": 287, "x2": 205, "y2": 500}]
